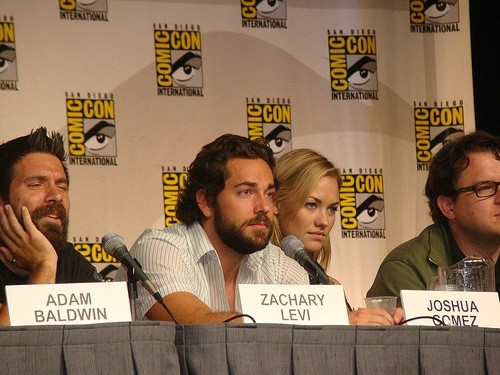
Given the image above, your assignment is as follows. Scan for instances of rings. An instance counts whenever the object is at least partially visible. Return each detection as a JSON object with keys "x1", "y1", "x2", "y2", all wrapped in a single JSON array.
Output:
[{"x1": 10, "y1": 259, "x2": 16, "y2": 265}]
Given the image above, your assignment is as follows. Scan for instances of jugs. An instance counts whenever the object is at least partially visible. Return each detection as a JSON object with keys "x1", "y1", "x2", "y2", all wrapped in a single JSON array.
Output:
[{"x1": 427, "y1": 256, "x2": 495, "y2": 297}]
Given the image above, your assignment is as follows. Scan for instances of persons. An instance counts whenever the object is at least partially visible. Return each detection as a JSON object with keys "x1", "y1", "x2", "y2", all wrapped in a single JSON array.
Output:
[
  {"x1": 270, "y1": 148, "x2": 406, "y2": 326},
  {"x1": 116, "y1": 133, "x2": 309, "y2": 324},
  {"x1": 365, "y1": 130, "x2": 500, "y2": 307},
  {"x1": 0, "y1": 127, "x2": 106, "y2": 326}
]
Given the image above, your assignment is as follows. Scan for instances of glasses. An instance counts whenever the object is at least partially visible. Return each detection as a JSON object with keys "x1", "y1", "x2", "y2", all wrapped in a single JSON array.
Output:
[{"x1": 456, "y1": 180, "x2": 500, "y2": 198}]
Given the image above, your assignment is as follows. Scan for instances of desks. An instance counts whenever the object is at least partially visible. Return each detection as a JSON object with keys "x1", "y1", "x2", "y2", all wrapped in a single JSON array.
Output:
[{"x1": 0, "y1": 323, "x2": 500, "y2": 375}]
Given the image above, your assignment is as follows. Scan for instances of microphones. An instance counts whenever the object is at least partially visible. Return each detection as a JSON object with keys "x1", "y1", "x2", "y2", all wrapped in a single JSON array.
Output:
[
  {"x1": 280, "y1": 235, "x2": 335, "y2": 285},
  {"x1": 103, "y1": 231, "x2": 163, "y2": 304}
]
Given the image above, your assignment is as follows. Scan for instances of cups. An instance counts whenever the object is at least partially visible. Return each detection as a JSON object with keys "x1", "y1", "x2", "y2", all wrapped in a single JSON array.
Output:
[{"x1": 364, "y1": 297, "x2": 398, "y2": 317}]
